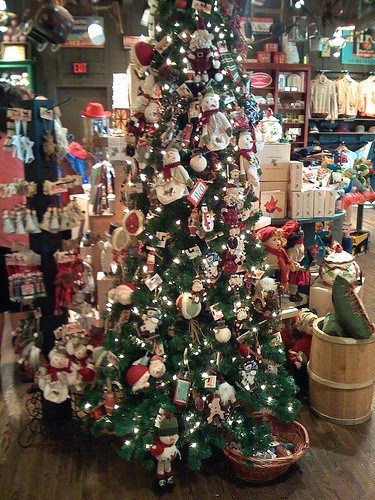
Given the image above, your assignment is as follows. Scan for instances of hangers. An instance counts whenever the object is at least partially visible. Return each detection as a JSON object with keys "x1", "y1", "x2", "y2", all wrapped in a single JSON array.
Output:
[
  {"x1": 319, "y1": 70, "x2": 327, "y2": 83},
  {"x1": 367, "y1": 72, "x2": 375, "y2": 83},
  {"x1": 339, "y1": 70, "x2": 353, "y2": 83}
]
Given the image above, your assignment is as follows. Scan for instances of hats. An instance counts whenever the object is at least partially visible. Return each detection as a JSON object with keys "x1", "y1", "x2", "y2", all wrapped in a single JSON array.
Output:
[
  {"x1": 80, "y1": 102, "x2": 111, "y2": 119},
  {"x1": 35, "y1": 96, "x2": 53, "y2": 120}
]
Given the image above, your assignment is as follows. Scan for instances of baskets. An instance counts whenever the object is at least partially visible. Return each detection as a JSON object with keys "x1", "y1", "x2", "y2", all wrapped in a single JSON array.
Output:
[{"x1": 223, "y1": 412, "x2": 310, "y2": 484}]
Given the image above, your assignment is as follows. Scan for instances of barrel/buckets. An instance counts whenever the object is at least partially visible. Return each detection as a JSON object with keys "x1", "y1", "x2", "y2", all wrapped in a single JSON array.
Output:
[{"x1": 306, "y1": 317, "x2": 375, "y2": 426}]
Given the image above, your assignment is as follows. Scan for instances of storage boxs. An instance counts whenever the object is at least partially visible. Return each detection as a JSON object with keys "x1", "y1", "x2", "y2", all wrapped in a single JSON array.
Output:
[
  {"x1": 254, "y1": 96, "x2": 263, "y2": 100},
  {"x1": 307, "y1": 275, "x2": 365, "y2": 317},
  {"x1": 342, "y1": 231, "x2": 371, "y2": 259},
  {"x1": 256, "y1": 41, "x2": 286, "y2": 65},
  {"x1": 256, "y1": 143, "x2": 336, "y2": 218},
  {"x1": 238, "y1": 16, "x2": 275, "y2": 42},
  {"x1": 284, "y1": 86, "x2": 290, "y2": 91},
  {"x1": 290, "y1": 86, "x2": 298, "y2": 91},
  {"x1": 297, "y1": 114, "x2": 304, "y2": 123},
  {"x1": 292, "y1": 119, "x2": 299, "y2": 122},
  {"x1": 266, "y1": 97, "x2": 275, "y2": 104},
  {"x1": 285, "y1": 112, "x2": 291, "y2": 121},
  {"x1": 290, "y1": 112, "x2": 296, "y2": 120},
  {"x1": 282, "y1": 118, "x2": 288, "y2": 123}
]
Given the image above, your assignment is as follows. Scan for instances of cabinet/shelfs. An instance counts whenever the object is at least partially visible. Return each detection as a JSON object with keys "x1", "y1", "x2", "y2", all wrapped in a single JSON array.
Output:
[
  {"x1": 309, "y1": 117, "x2": 375, "y2": 141},
  {"x1": 0, "y1": 58, "x2": 39, "y2": 98},
  {"x1": 248, "y1": 0, "x2": 285, "y2": 23},
  {"x1": 242, "y1": 62, "x2": 312, "y2": 148}
]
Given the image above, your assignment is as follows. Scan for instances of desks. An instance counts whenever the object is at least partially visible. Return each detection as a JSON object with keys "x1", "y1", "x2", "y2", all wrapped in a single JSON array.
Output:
[
  {"x1": 269, "y1": 206, "x2": 346, "y2": 270},
  {"x1": 338, "y1": 191, "x2": 375, "y2": 230},
  {"x1": 279, "y1": 293, "x2": 309, "y2": 330},
  {"x1": 96, "y1": 270, "x2": 117, "y2": 322}
]
